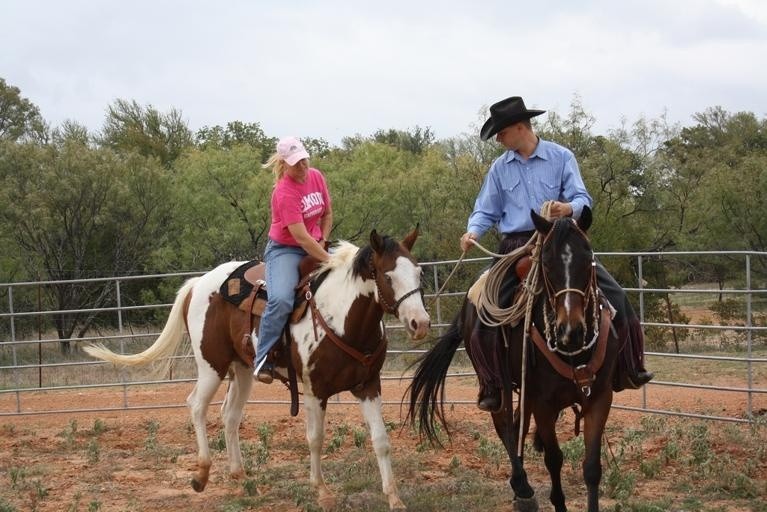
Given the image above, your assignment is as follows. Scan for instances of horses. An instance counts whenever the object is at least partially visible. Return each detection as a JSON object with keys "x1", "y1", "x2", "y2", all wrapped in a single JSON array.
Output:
[
  {"x1": 397, "y1": 205, "x2": 622, "y2": 512},
  {"x1": 81, "y1": 220, "x2": 432, "y2": 512}
]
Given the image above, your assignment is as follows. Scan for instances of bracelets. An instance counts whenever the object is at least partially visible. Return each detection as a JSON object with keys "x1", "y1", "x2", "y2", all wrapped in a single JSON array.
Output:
[{"x1": 320, "y1": 237, "x2": 327, "y2": 242}]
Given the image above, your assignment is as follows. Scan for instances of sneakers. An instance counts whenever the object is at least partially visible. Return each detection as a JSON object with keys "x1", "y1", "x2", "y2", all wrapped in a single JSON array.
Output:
[
  {"x1": 613, "y1": 371, "x2": 655, "y2": 392},
  {"x1": 257, "y1": 369, "x2": 273, "y2": 383},
  {"x1": 479, "y1": 395, "x2": 512, "y2": 413}
]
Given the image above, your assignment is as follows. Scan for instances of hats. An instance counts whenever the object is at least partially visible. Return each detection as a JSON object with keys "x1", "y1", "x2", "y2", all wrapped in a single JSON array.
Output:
[
  {"x1": 277, "y1": 136, "x2": 309, "y2": 166},
  {"x1": 480, "y1": 97, "x2": 546, "y2": 142}
]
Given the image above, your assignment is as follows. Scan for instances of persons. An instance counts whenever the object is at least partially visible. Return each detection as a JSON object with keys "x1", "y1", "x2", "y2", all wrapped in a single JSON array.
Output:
[
  {"x1": 253, "y1": 138, "x2": 333, "y2": 384},
  {"x1": 461, "y1": 96, "x2": 655, "y2": 411}
]
